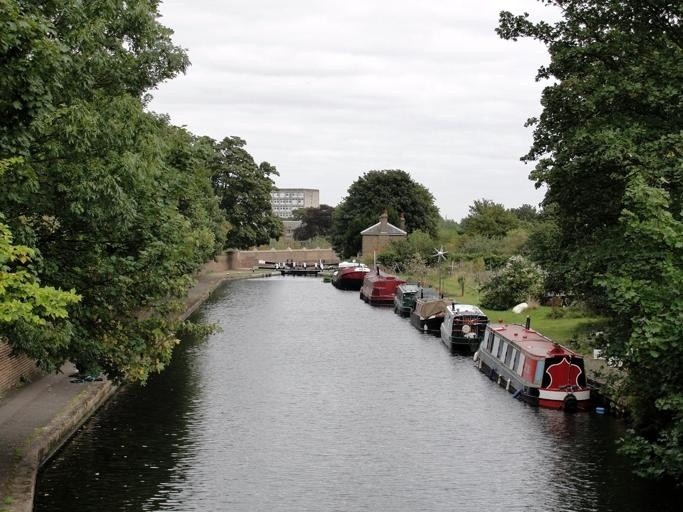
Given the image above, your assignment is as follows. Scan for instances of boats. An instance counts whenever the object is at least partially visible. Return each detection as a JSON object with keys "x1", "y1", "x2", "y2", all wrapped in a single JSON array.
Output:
[
  {"x1": 320, "y1": 256, "x2": 445, "y2": 337},
  {"x1": 464, "y1": 314, "x2": 595, "y2": 415},
  {"x1": 438, "y1": 301, "x2": 490, "y2": 357}
]
